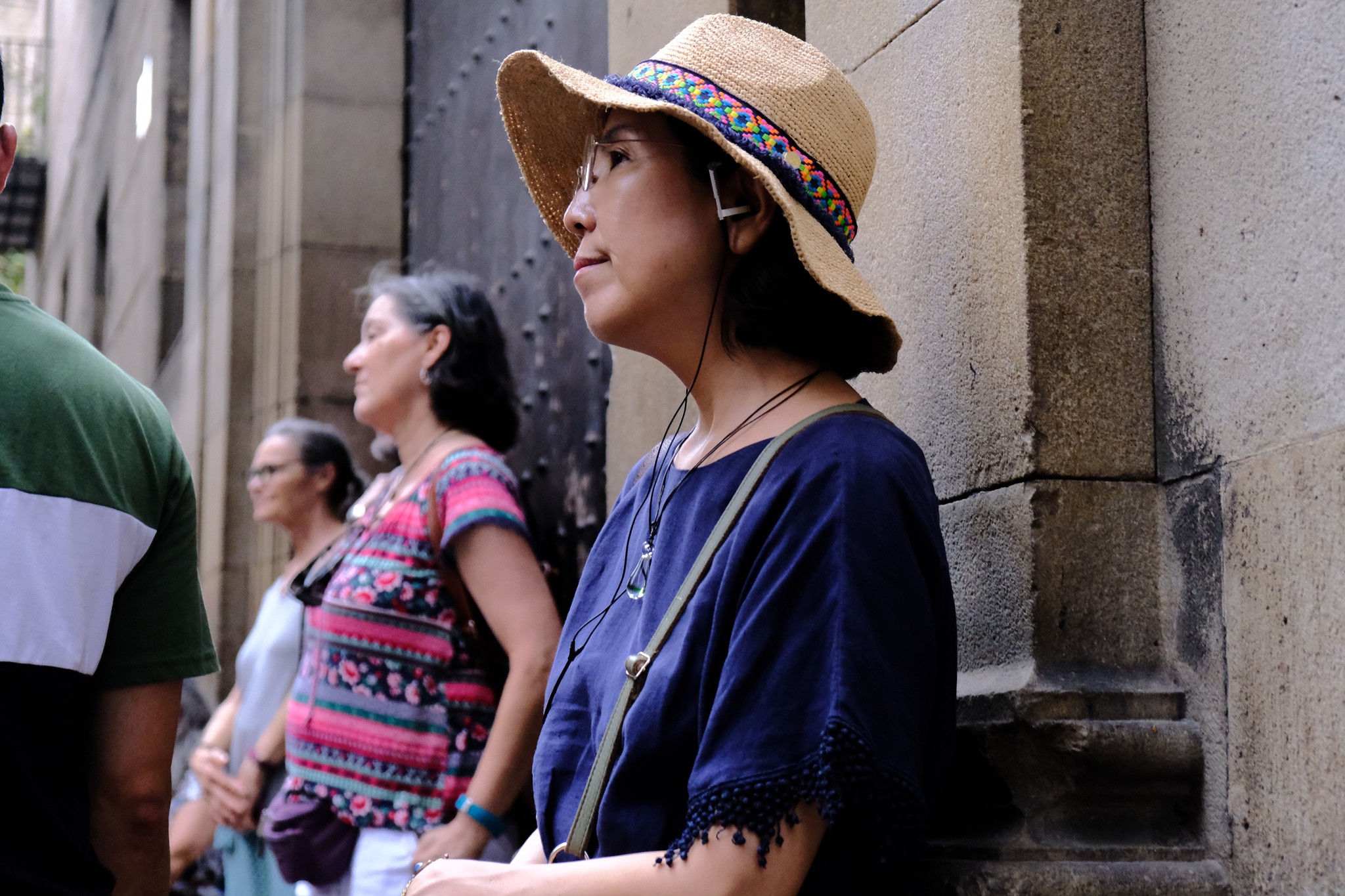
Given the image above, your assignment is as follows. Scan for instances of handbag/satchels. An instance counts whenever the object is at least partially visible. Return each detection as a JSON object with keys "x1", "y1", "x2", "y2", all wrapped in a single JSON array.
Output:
[{"x1": 262, "y1": 789, "x2": 361, "y2": 886}]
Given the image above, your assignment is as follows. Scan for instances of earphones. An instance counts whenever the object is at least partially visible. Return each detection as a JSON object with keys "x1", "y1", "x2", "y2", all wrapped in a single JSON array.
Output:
[{"x1": 719, "y1": 201, "x2": 752, "y2": 222}]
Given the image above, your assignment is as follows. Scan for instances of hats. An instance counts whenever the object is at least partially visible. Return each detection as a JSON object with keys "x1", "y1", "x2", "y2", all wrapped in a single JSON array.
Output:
[{"x1": 495, "y1": 13, "x2": 903, "y2": 380}]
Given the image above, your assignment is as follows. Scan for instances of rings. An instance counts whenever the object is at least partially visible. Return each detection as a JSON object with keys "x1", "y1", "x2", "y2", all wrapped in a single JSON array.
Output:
[{"x1": 413, "y1": 860, "x2": 431, "y2": 874}]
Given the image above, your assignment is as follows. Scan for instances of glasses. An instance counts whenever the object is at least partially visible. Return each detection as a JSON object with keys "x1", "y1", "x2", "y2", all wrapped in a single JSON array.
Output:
[
  {"x1": 570, "y1": 130, "x2": 682, "y2": 196},
  {"x1": 288, "y1": 523, "x2": 368, "y2": 608},
  {"x1": 240, "y1": 458, "x2": 303, "y2": 485}
]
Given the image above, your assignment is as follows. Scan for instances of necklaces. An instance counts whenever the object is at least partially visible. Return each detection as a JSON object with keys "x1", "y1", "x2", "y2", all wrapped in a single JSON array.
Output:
[
  {"x1": 628, "y1": 371, "x2": 820, "y2": 599},
  {"x1": 371, "y1": 426, "x2": 451, "y2": 523}
]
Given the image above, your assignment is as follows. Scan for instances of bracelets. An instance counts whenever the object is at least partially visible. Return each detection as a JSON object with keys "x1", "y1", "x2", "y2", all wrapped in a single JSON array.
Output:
[
  {"x1": 455, "y1": 793, "x2": 506, "y2": 841},
  {"x1": 246, "y1": 748, "x2": 276, "y2": 775}
]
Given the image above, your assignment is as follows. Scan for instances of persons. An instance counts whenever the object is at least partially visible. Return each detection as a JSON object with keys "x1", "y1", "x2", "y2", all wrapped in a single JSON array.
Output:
[
  {"x1": 0, "y1": 52, "x2": 221, "y2": 896},
  {"x1": 398, "y1": 11, "x2": 960, "y2": 895},
  {"x1": 145, "y1": 416, "x2": 369, "y2": 896},
  {"x1": 285, "y1": 257, "x2": 563, "y2": 895}
]
